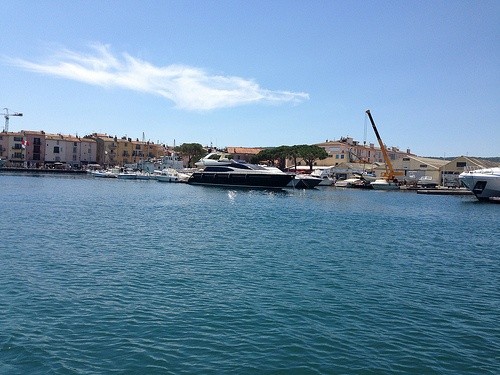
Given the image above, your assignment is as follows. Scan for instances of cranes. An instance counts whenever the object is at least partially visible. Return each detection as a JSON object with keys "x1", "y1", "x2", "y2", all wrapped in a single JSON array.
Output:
[{"x1": 0, "y1": 108, "x2": 24, "y2": 131}]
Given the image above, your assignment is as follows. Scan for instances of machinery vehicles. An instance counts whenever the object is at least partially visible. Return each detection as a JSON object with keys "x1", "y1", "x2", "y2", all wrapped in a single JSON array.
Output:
[{"x1": 364, "y1": 109, "x2": 404, "y2": 182}]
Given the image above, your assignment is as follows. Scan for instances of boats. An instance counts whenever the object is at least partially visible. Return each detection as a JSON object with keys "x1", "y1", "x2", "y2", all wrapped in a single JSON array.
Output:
[
  {"x1": 187, "y1": 154, "x2": 296, "y2": 190},
  {"x1": 94, "y1": 166, "x2": 189, "y2": 183},
  {"x1": 312, "y1": 169, "x2": 400, "y2": 190},
  {"x1": 287, "y1": 167, "x2": 324, "y2": 189},
  {"x1": 458, "y1": 167, "x2": 500, "y2": 203}
]
